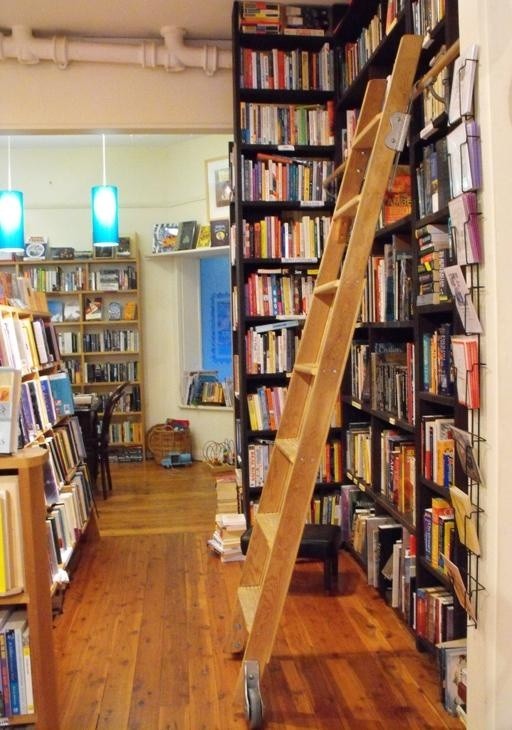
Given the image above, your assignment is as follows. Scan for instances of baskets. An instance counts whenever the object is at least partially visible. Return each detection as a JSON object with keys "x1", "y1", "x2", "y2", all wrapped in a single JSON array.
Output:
[{"x1": 151, "y1": 429, "x2": 192, "y2": 465}]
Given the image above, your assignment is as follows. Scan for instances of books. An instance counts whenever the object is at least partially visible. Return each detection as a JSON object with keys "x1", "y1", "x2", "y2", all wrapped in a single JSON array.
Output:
[
  {"x1": 24, "y1": 266, "x2": 142, "y2": 444},
  {"x1": 206, "y1": 512, "x2": 247, "y2": 564},
  {"x1": 186, "y1": 370, "x2": 234, "y2": 407},
  {"x1": 0, "y1": 270, "x2": 91, "y2": 718},
  {"x1": 153, "y1": 221, "x2": 229, "y2": 254},
  {"x1": 238, "y1": 0, "x2": 481, "y2": 718}
]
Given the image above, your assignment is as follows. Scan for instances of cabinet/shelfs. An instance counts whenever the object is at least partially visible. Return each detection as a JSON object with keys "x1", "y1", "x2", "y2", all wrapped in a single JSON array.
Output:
[
  {"x1": 0, "y1": 232, "x2": 148, "y2": 465},
  {"x1": 345, "y1": 0, "x2": 511, "y2": 729},
  {"x1": 229, "y1": 1, "x2": 345, "y2": 523},
  {"x1": 0, "y1": 304, "x2": 103, "y2": 730}
]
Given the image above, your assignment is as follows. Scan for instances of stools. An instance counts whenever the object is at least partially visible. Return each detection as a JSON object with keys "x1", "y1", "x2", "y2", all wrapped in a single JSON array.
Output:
[{"x1": 240, "y1": 524, "x2": 342, "y2": 596}]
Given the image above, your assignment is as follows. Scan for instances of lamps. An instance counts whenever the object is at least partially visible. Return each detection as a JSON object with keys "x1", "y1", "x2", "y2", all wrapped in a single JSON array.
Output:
[
  {"x1": 0, "y1": 134, "x2": 26, "y2": 256},
  {"x1": 89, "y1": 134, "x2": 121, "y2": 250}
]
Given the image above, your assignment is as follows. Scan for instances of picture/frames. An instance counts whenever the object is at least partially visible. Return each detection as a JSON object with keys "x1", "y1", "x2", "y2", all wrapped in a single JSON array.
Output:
[
  {"x1": 91, "y1": 245, "x2": 116, "y2": 260},
  {"x1": 113, "y1": 230, "x2": 138, "y2": 260},
  {"x1": 203, "y1": 154, "x2": 229, "y2": 223}
]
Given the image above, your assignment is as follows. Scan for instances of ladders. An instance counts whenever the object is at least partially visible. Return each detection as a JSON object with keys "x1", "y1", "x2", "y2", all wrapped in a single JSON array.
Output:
[{"x1": 222, "y1": 34, "x2": 424, "y2": 730}]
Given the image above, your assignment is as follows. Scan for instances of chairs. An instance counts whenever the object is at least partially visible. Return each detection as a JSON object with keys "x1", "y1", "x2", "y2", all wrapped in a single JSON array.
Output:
[{"x1": 85, "y1": 379, "x2": 131, "y2": 501}]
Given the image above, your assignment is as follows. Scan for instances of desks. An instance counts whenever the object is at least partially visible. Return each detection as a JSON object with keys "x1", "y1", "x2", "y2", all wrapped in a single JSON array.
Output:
[{"x1": 73, "y1": 396, "x2": 103, "y2": 492}]
